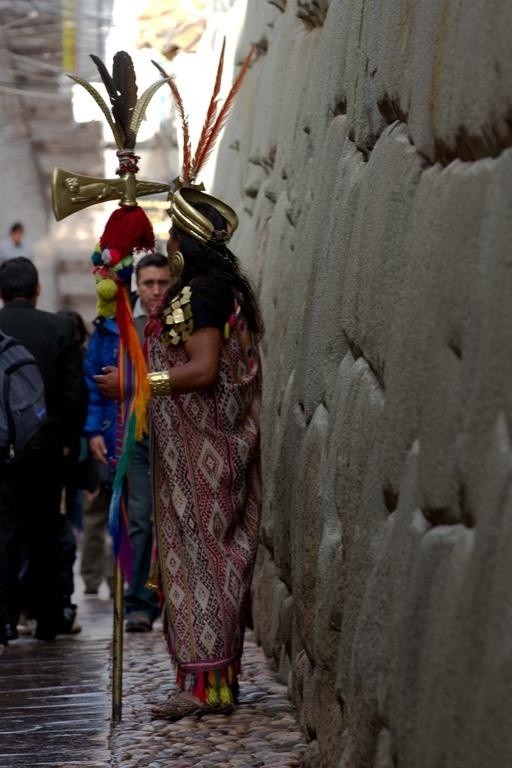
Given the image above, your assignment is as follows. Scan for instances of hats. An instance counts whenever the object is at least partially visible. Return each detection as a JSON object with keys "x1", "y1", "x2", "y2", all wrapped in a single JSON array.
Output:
[{"x1": 172, "y1": 186, "x2": 237, "y2": 244}]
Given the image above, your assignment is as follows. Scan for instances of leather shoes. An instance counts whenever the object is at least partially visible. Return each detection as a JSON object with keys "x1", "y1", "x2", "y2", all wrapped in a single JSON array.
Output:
[
  {"x1": 35, "y1": 624, "x2": 81, "y2": 641},
  {"x1": 125, "y1": 617, "x2": 151, "y2": 632}
]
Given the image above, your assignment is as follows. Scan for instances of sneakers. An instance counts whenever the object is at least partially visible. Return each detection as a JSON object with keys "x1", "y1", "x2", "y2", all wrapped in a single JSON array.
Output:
[{"x1": 150, "y1": 694, "x2": 233, "y2": 719}]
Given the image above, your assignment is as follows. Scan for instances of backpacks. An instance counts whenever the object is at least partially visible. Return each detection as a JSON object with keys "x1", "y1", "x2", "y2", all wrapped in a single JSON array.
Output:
[{"x1": 0, "y1": 329, "x2": 46, "y2": 463}]
